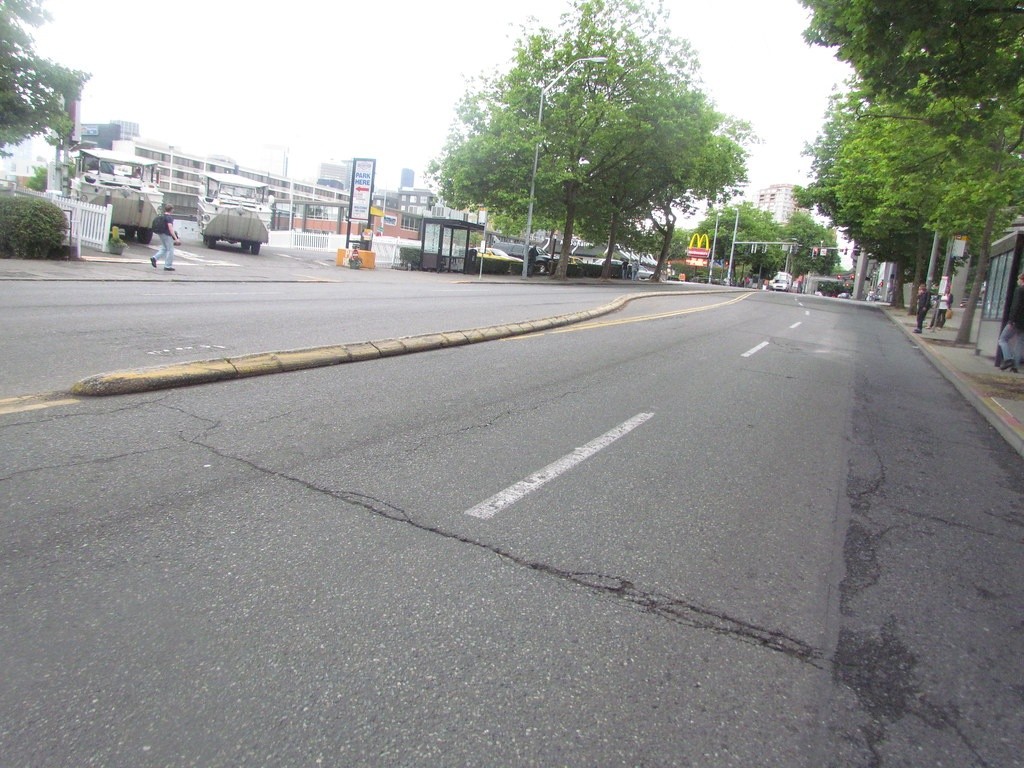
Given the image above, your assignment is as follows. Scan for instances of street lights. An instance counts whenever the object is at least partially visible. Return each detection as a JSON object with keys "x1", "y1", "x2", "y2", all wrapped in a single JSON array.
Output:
[
  {"x1": 725, "y1": 207, "x2": 741, "y2": 286},
  {"x1": 521, "y1": 56, "x2": 608, "y2": 282},
  {"x1": 709, "y1": 212, "x2": 723, "y2": 284}
]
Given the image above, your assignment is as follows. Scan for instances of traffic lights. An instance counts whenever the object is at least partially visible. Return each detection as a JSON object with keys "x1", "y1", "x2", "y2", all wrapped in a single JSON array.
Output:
[
  {"x1": 850, "y1": 274, "x2": 855, "y2": 280},
  {"x1": 837, "y1": 274, "x2": 841, "y2": 279},
  {"x1": 881, "y1": 282, "x2": 883, "y2": 287},
  {"x1": 725, "y1": 260, "x2": 729, "y2": 266},
  {"x1": 846, "y1": 282, "x2": 850, "y2": 287},
  {"x1": 812, "y1": 247, "x2": 818, "y2": 259}
]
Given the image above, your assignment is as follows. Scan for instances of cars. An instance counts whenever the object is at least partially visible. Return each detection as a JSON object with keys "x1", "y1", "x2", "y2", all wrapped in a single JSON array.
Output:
[
  {"x1": 627, "y1": 264, "x2": 655, "y2": 280},
  {"x1": 495, "y1": 242, "x2": 584, "y2": 275},
  {"x1": 594, "y1": 259, "x2": 624, "y2": 267},
  {"x1": 471, "y1": 247, "x2": 523, "y2": 263}
]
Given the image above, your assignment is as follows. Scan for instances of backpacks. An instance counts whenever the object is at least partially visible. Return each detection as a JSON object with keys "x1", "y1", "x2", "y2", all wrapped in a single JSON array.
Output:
[{"x1": 152, "y1": 214, "x2": 172, "y2": 233}]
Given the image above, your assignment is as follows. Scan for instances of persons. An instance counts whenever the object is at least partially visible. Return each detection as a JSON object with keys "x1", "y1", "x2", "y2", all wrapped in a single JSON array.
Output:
[
  {"x1": 998, "y1": 272, "x2": 1024, "y2": 373},
  {"x1": 150, "y1": 203, "x2": 181, "y2": 271},
  {"x1": 527, "y1": 245, "x2": 538, "y2": 277},
  {"x1": 925, "y1": 284, "x2": 953, "y2": 331},
  {"x1": 631, "y1": 259, "x2": 639, "y2": 281},
  {"x1": 797, "y1": 273, "x2": 804, "y2": 293},
  {"x1": 912, "y1": 284, "x2": 931, "y2": 333},
  {"x1": 622, "y1": 258, "x2": 629, "y2": 281},
  {"x1": 134, "y1": 167, "x2": 141, "y2": 178},
  {"x1": 744, "y1": 277, "x2": 750, "y2": 288}
]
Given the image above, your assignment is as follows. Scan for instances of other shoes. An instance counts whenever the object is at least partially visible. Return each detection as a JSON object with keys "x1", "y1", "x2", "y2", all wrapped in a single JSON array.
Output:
[
  {"x1": 1000, "y1": 360, "x2": 1013, "y2": 369},
  {"x1": 913, "y1": 328, "x2": 922, "y2": 333},
  {"x1": 1010, "y1": 367, "x2": 1017, "y2": 373},
  {"x1": 164, "y1": 266, "x2": 175, "y2": 271},
  {"x1": 150, "y1": 256, "x2": 156, "y2": 268}
]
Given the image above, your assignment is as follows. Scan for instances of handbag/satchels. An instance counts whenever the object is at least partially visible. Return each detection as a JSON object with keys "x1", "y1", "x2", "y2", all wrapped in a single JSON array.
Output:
[{"x1": 946, "y1": 309, "x2": 952, "y2": 319}]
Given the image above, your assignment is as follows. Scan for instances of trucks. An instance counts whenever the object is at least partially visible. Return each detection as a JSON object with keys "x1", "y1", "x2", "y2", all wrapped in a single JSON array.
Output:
[
  {"x1": 769, "y1": 273, "x2": 803, "y2": 293},
  {"x1": 197, "y1": 171, "x2": 272, "y2": 254},
  {"x1": 74, "y1": 151, "x2": 164, "y2": 244}
]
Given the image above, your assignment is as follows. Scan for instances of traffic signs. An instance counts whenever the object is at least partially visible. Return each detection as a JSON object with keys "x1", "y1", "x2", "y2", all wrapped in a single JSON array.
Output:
[{"x1": 350, "y1": 158, "x2": 374, "y2": 225}]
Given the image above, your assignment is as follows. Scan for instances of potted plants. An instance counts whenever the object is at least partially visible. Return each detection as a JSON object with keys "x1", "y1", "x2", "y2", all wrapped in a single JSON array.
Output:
[
  {"x1": 106, "y1": 238, "x2": 129, "y2": 255},
  {"x1": 348, "y1": 257, "x2": 363, "y2": 269}
]
